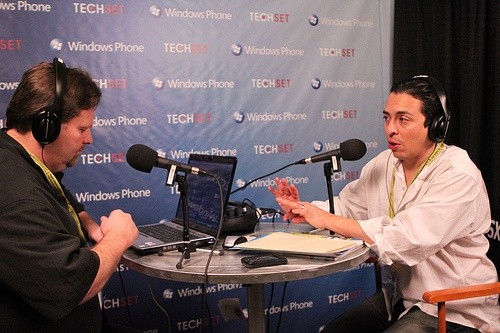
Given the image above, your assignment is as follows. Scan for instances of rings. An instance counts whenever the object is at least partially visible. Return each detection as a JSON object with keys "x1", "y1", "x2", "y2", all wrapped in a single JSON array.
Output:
[{"x1": 302, "y1": 203, "x2": 305, "y2": 210}]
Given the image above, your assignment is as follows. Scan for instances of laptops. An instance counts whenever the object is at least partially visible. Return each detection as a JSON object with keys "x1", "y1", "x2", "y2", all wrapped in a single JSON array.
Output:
[{"x1": 128, "y1": 155, "x2": 238, "y2": 255}]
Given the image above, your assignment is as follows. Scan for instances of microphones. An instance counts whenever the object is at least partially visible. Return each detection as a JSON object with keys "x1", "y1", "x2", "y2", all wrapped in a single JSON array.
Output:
[
  {"x1": 299, "y1": 139, "x2": 367, "y2": 164},
  {"x1": 126, "y1": 144, "x2": 205, "y2": 176}
]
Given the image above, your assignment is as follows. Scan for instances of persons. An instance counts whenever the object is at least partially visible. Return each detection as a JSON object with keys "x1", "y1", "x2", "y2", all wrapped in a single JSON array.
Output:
[
  {"x1": 0, "y1": 60, "x2": 139, "y2": 333},
  {"x1": 268, "y1": 75, "x2": 500, "y2": 333}
]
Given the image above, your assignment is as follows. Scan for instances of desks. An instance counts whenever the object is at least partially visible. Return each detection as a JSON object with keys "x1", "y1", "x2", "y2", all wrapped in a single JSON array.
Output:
[{"x1": 121, "y1": 222, "x2": 371, "y2": 333}]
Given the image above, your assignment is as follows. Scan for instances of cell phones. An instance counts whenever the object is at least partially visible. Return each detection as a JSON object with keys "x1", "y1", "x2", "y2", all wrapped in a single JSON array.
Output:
[{"x1": 241, "y1": 254, "x2": 287, "y2": 269}]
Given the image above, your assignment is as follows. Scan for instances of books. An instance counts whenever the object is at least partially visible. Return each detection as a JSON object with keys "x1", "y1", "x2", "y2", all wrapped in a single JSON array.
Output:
[{"x1": 231, "y1": 232, "x2": 364, "y2": 261}]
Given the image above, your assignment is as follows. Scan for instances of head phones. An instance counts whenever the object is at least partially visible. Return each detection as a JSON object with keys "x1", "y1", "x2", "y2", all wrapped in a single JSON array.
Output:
[
  {"x1": 415, "y1": 75, "x2": 452, "y2": 142},
  {"x1": 30, "y1": 57, "x2": 67, "y2": 145}
]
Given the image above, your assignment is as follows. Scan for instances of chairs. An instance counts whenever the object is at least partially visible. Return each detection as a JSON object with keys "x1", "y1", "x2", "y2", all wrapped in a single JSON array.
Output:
[{"x1": 363, "y1": 255, "x2": 500, "y2": 333}]
